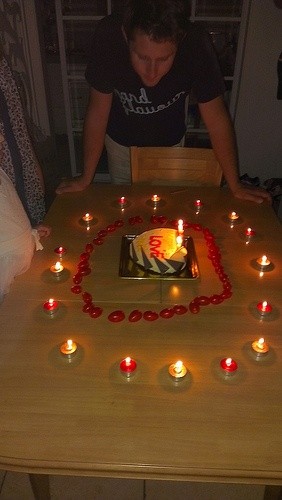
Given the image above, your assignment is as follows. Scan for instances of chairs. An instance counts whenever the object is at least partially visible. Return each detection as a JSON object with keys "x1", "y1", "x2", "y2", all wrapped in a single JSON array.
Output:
[{"x1": 129, "y1": 145, "x2": 223, "y2": 186}]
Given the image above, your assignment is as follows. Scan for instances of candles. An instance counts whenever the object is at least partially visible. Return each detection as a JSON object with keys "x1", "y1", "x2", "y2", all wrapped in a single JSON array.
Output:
[
  {"x1": 177, "y1": 218, "x2": 185, "y2": 244},
  {"x1": 256, "y1": 300, "x2": 273, "y2": 316},
  {"x1": 255, "y1": 255, "x2": 271, "y2": 269},
  {"x1": 251, "y1": 338, "x2": 271, "y2": 357},
  {"x1": 244, "y1": 227, "x2": 255, "y2": 237},
  {"x1": 192, "y1": 199, "x2": 202, "y2": 209},
  {"x1": 167, "y1": 360, "x2": 188, "y2": 382},
  {"x1": 119, "y1": 356, "x2": 137, "y2": 378},
  {"x1": 228, "y1": 211, "x2": 240, "y2": 222},
  {"x1": 82, "y1": 213, "x2": 96, "y2": 226},
  {"x1": 220, "y1": 357, "x2": 239, "y2": 376},
  {"x1": 49, "y1": 261, "x2": 64, "y2": 276},
  {"x1": 42, "y1": 298, "x2": 59, "y2": 314},
  {"x1": 54, "y1": 246, "x2": 65, "y2": 258},
  {"x1": 150, "y1": 194, "x2": 161, "y2": 205},
  {"x1": 116, "y1": 196, "x2": 129, "y2": 208},
  {"x1": 60, "y1": 339, "x2": 77, "y2": 359}
]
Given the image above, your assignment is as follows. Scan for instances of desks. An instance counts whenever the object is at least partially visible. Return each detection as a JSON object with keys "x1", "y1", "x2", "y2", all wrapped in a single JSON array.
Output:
[{"x1": 0, "y1": 185, "x2": 282, "y2": 500}]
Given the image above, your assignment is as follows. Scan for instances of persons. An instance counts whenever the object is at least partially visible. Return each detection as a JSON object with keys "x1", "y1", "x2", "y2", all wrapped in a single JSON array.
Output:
[
  {"x1": 0, "y1": 51, "x2": 52, "y2": 304},
  {"x1": 55, "y1": 0, "x2": 272, "y2": 205}
]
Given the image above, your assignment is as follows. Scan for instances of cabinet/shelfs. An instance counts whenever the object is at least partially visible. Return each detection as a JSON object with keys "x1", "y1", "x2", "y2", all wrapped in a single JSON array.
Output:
[{"x1": 55, "y1": 0, "x2": 250, "y2": 176}]
